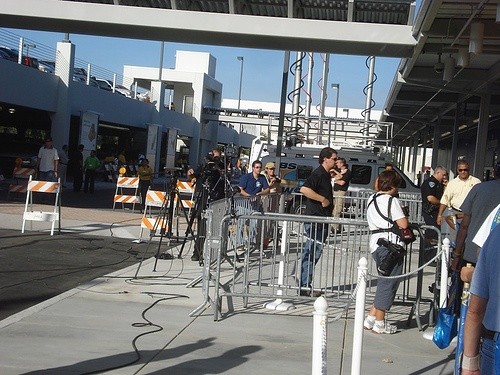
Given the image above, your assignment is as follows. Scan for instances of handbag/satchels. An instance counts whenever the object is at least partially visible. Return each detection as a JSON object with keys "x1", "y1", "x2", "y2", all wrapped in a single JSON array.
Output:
[
  {"x1": 390, "y1": 221, "x2": 417, "y2": 244},
  {"x1": 432, "y1": 307, "x2": 458, "y2": 349},
  {"x1": 371, "y1": 238, "x2": 406, "y2": 277}
]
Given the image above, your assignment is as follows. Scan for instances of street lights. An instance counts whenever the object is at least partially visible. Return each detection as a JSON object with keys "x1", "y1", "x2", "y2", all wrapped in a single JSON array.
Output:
[
  {"x1": 343, "y1": 109, "x2": 349, "y2": 141},
  {"x1": 330, "y1": 84, "x2": 339, "y2": 140},
  {"x1": 236, "y1": 56, "x2": 244, "y2": 117}
]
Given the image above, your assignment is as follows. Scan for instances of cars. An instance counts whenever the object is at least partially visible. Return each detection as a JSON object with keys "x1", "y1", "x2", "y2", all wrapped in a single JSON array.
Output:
[{"x1": 0, "y1": 48, "x2": 139, "y2": 99}]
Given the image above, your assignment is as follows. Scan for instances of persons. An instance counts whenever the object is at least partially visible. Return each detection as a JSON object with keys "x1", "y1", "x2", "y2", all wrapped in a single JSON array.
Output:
[
  {"x1": 296, "y1": 147, "x2": 339, "y2": 299},
  {"x1": 455, "y1": 213, "x2": 458, "y2": 219},
  {"x1": 461, "y1": 221, "x2": 500, "y2": 375},
  {"x1": 363, "y1": 169, "x2": 409, "y2": 336},
  {"x1": 331, "y1": 157, "x2": 351, "y2": 236},
  {"x1": 449, "y1": 161, "x2": 500, "y2": 318},
  {"x1": 459, "y1": 203, "x2": 500, "y2": 291},
  {"x1": 452, "y1": 213, "x2": 463, "y2": 219},
  {"x1": 374, "y1": 163, "x2": 394, "y2": 192},
  {"x1": 30, "y1": 137, "x2": 283, "y2": 268},
  {"x1": 436, "y1": 160, "x2": 482, "y2": 258},
  {"x1": 420, "y1": 165, "x2": 449, "y2": 267}
]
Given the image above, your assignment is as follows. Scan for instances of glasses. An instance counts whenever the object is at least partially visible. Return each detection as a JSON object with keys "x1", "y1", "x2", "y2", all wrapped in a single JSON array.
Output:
[
  {"x1": 268, "y1": 167, "x2": 276, "y2": 171},
  {"x1": 45, "y1": 140, "x2": 51, "y2": 142},
  {"x1": 255, "y1": 166, "x2": 262, "y2": 169},
  {"x1": 330, "y1": 157, "x2": 338, "y2": 161},
  {"x1": 456, "y1": 168, "x2": 469, "y2": 173}
]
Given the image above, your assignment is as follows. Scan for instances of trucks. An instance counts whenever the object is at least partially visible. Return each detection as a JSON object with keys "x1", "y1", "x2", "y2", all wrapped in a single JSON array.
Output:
[{"x1": 246, "y1": 137, "x2": 420, "y2": 217}]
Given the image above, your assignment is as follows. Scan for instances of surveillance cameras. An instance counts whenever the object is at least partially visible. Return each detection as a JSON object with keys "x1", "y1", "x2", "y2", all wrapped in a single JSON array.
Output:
[{"x1": 434, "y1": 62, "x2": 444, "y2": 74}]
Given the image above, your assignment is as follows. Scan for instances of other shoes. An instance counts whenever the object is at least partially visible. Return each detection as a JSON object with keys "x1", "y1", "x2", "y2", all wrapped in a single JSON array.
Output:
[
  {"x1": 191, "y1": 253, "x2": 199, "y2": 261},
  {"x1": 297, "y1": 285, "x2": 323, "y2": 297},
  {"x1": 330, "y1": 226, "x2": 345, "y2": 234},
  {"x1": 263, "y1": 239, "x2": 269, "y2": 249}
]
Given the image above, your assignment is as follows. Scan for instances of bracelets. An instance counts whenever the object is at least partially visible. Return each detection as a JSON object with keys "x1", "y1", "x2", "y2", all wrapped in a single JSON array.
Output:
[
  {"x1": 454, "y1": 252, "x2": 461, "y2": 256},
  {"x1": 462, "y1": 353, "x2": 481, "y2": 371},
  {"x1": 437, "y1": 216, "x2": 442, "y2": 218}
]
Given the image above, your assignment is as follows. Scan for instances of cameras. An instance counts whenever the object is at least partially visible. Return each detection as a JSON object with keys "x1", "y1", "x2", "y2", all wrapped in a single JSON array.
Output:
[{"x1": 376, "y1": 238, "x2": 405, "y2": 276}]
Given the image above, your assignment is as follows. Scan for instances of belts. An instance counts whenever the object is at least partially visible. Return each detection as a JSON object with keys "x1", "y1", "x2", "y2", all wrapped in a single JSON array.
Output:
[{"x1": 451, "y1": 205, "x2": 462, "y2": 212}]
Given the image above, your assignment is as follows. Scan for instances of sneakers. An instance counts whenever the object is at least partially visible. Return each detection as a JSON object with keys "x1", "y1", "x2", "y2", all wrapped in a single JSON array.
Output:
[
  {"x1": 372, "y1": 320, "x2": 397, "y2": 334},
  {"x1": 363, "y1": 314, "x2": 376, "y2": 329}
]
